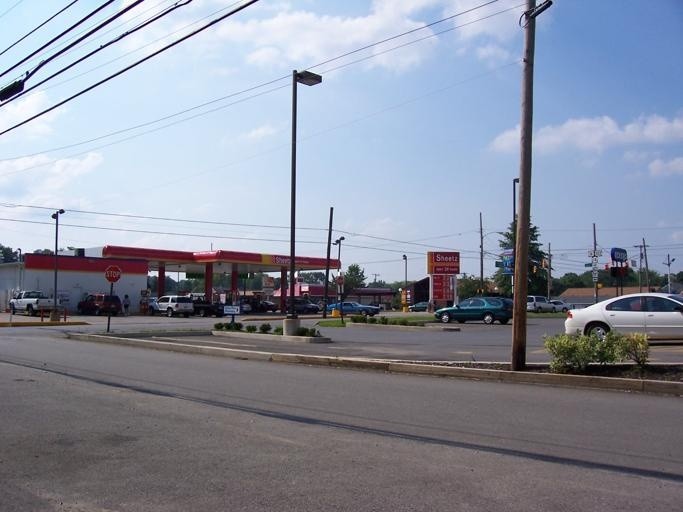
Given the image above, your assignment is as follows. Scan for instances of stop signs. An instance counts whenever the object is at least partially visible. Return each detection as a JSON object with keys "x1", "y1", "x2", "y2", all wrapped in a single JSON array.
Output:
[{"x1": 104, "y1": 265, "x2": 121, "y2": 282}]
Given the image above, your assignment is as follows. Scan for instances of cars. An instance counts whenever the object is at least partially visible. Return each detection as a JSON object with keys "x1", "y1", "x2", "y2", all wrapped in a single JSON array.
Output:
[
  {"x1": 564, "y1": 292, "x2": 682, "y2": 343},
  {"x1": 408, "y1": 295, "x2": 571, "y2": 324},
  {"x1": 78, "y1": 293, "x2": 122, "y2": 316},
  {"x1": 149, "y1": 291, "x2": 380, "y2": 317}
]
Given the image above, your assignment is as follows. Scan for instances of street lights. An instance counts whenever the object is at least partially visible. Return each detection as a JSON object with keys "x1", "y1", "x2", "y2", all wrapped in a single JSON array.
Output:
[
  {"x1": 513, "y1": 179, "x2": 519, "y2": 266},
  {"x1": 402, "y1": 255, "x2": 407, "y2": 286},
  {"x1": 332, "y1": 237, "x2": 344, "y2": 272},
  {"x1": 50, "y1": 209, "x2": 64, "y2": 321},
  {"x1": 285, "y1": 70, "x2": 321, "y2": 319}
]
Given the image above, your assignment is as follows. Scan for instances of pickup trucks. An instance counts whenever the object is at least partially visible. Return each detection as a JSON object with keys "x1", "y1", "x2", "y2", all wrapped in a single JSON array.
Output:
[{"x1": 9, "y1": 290, "x2": 63, "y2": 316}]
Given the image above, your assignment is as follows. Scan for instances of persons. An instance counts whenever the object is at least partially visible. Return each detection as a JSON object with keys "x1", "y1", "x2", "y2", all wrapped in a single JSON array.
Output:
[{"x1": 121, "y1": 294, "x2": 131, "y2": 316}]
